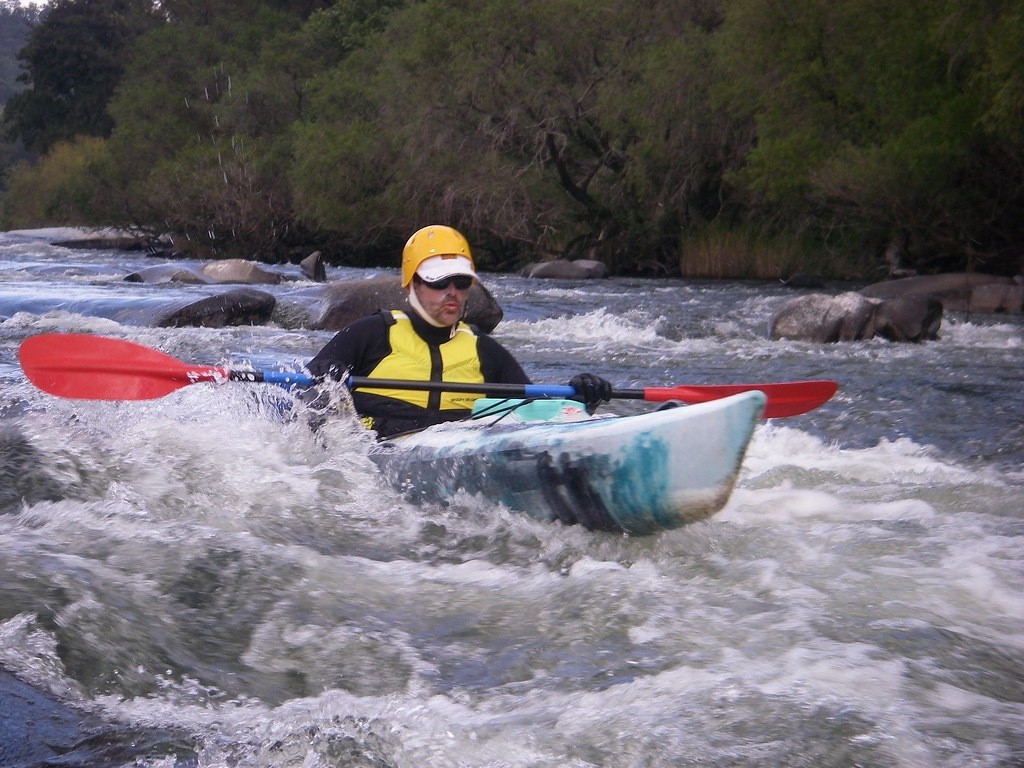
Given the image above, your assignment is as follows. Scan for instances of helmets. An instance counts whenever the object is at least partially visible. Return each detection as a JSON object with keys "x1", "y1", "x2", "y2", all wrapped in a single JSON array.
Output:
[{"x1": 401, "y1": 225, "x2": 475, "y2": 290}]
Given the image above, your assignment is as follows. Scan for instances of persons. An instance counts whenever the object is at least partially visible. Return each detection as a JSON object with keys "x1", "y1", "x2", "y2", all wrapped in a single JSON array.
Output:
[{"x1": 295, "y1": 224, "x2": 613, "y2": 448}]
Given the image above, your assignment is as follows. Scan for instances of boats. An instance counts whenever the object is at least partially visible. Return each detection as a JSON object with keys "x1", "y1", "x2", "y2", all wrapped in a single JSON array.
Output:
[{"x1": 346, "y1": 385, "x2": 771, "y2": 546}]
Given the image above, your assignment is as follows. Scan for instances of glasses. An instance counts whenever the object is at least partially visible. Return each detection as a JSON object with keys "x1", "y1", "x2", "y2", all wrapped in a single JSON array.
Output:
[{"x1": 412, "y1": 276, "x2": 473, "y2": 290}]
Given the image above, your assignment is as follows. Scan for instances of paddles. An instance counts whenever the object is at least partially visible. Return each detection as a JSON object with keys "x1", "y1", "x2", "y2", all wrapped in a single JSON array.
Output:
[{"x1": 16, "y1": 332, "x2": 837, "y2": 420}]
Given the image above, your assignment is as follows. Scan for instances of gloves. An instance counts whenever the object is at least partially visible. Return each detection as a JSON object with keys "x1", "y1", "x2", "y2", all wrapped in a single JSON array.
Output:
[
  {"x1": 566, "y1": 372, "x2": 612, "y2": 417},
  {"x1": 307, "y1": 360, "x2": 348, "y2": 386}
]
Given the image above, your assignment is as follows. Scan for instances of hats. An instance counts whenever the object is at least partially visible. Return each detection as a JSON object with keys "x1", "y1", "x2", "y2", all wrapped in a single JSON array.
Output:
[{"x1": 414, "y1": 255, "x2": 482, "y2": 283}]
